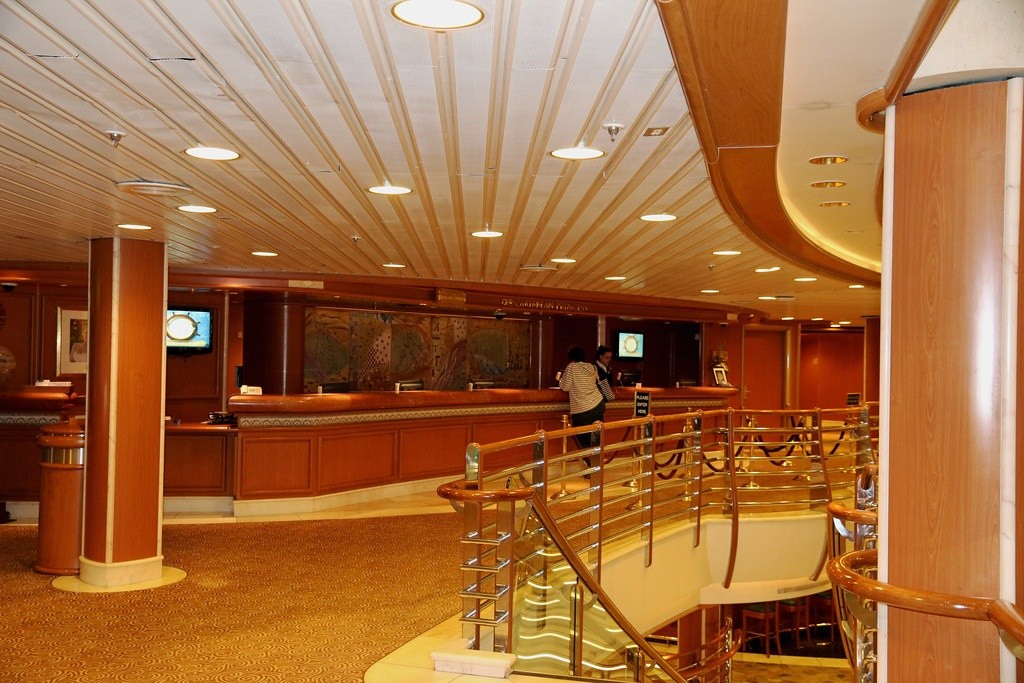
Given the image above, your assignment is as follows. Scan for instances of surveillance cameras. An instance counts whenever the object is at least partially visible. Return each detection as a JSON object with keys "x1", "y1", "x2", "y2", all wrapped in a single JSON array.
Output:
[{"x1": 493, "y1": 312, "x2": 507, "y2": 320}]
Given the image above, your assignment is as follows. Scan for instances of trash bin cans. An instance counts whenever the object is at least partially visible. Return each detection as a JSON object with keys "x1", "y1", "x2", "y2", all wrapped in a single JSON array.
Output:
[{"x1": 30, "y1": 424, "x2": 87, "y2": 576}]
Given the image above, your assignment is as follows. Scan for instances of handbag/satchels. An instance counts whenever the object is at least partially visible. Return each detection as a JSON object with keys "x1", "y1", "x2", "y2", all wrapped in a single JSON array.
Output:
[{"x1": 598, "y1": 378, "x2": 616, "y2": 402}]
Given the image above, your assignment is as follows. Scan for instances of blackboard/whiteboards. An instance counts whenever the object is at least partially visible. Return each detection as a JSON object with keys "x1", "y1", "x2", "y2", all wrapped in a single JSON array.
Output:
[{"x1": 634, "y1": 390, "x2": 651, "y2": 417}]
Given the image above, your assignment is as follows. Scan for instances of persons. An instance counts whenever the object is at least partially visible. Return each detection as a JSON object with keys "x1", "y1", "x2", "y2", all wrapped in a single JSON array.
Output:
[
  {"x1": 594, "y1": 346, "x2": 622, "y2": 387},
  {"x1": 559, "y1": 345, "x2": 604, "y2": 480}
]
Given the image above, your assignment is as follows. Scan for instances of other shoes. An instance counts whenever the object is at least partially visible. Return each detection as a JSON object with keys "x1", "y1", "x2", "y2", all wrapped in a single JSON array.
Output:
[{"x1": 583, "y1": 475, "x2": 591, "y2": 478}]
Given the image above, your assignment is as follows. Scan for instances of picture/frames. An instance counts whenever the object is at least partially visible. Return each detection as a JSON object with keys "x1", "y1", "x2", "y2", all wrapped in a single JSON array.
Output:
[
  {"x1": 712, "y1": 367, "x2": 727, "y2": 386},
  {"x1": 55, "y1": 304, "x2": 88, "y2": 380}
]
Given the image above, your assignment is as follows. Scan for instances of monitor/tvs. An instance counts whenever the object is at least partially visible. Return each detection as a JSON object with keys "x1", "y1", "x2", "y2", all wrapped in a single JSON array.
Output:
[
  {"x1": 616, "y1": 331, "x2": 646, "y2": 362},
  {"x1": 471, "y1": 379, "x2": 495, "y2": 389},
  {"x1": 165, "y1": 307, "x2": 214, "y2": 353},
  {"x1": 616, "y1": 373, "x2": 641, "y2": 387},
  {"x1": 397, "y1": 380, "x2": 424, "y2": 392},
  {"x1": 320, "y1": 382, "x2": 349, "y2": 394}
]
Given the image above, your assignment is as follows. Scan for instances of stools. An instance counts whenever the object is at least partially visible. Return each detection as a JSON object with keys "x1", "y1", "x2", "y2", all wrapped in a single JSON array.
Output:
[{"x1": 741, "y1": 589, "x2": 836, "y2": 659}]
